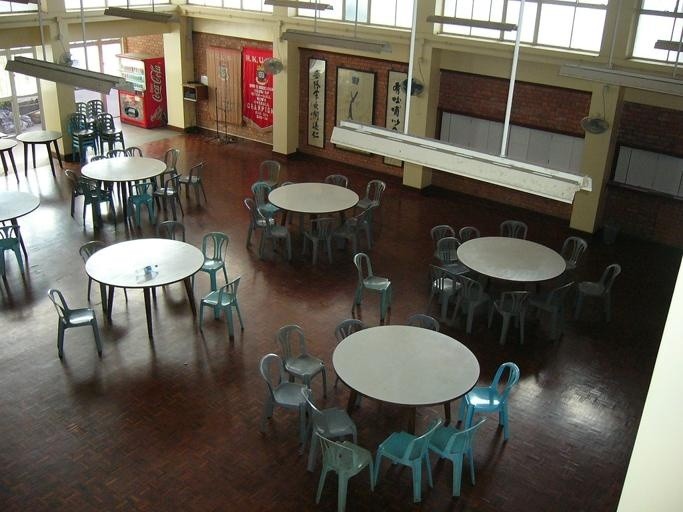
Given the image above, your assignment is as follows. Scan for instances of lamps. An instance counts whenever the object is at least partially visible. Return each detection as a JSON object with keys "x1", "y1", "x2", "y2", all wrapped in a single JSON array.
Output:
[
  {"x1": 264, "y1": 0, "x2": 334, "y2": 10},
  {"x1": 5, "y1": 56, "x2": 134, "y2": 95},
  {"x1": 322, "y1": 2, "x2": 603, "y2": 211},
  {"x1": 104, "y1": 7, "x2": 180, "y2": 24},
  {"x1": 655, "y1": 40, "x2": 683, "y2": 52},
  {"x1": 426, "y1": 15, "x2": 518, "y2": 31},
  {"x1": 0, "y1": 0, "x2": 38, "y2": 4},
  {"x1": 581, "y1": 100, "x2": 611, "y2": 136}
]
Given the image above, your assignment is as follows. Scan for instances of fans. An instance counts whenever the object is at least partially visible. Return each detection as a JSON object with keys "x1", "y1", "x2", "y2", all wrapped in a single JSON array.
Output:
[
  {"x1": 263, "y1": 58, "x2": 283, "y2": 75},
  {"x1": 59, "y1": 52, "x2": 78, "y2": 67},
  {"x1": 401, "y1": 77, "x2": 424, "y2": 97}
]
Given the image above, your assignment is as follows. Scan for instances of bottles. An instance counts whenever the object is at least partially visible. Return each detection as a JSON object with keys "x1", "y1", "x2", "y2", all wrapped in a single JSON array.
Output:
[
  {"x1": 119, "y1": 66, "x2": 145, "y2": 91},
  {"x1": 136, "y1": 271, "x2": 159, "y2": 283},
  {"x1": 136, "y1": 263, "x2": 158, "y2": 276}
]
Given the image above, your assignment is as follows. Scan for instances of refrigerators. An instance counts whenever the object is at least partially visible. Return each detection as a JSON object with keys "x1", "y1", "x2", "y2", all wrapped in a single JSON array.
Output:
[{"x1": 113, "y1": 52, "x2": 167, "y2": 130}]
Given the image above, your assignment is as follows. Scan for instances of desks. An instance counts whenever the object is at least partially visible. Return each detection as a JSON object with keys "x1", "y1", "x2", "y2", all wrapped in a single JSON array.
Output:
[
  {"x1": 1, "y1": 138, "x2": 22, "y2": 185},
  {"x1": 268, "y1": 181, "x2": 358, "y2": 223},
  {"x1": 16, "y1": 130, "x2": 64, "y2": 178},
  {"x1": 328, "y1": 323, "x2": 479, "y2": 427},
  {"x1": 85, "y1": 236, "x2": 200, "y2": 338},
  {"x1": 1, "y1": 190, "x2": 43, "y2": 257},
  {"x1": 83, "y1": 159, "x2": 167, "y2": 198},
  {"x1": 456, "y1": 235, "x2": 571, "y2": 297}
]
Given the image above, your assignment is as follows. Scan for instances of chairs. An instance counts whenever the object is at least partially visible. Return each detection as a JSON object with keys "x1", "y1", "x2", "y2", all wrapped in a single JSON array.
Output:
[
  {"x1": 1, "y1": 226, "x2": 24, "y2": 278},
  {"x1": 108, "y1": 149, "x2": 125, "y2": 158},
  {"x1": 500, "y1": 219, "x2": 527, "y2": 240},
  {"x1": 436, "y1": 239, "x2": 474, "y2": 276},
  {"x1": 256, "y1": 208, "x2": 293, "y2": 264},
  {"x1": 75, "y1": 239, "x2": 128, "y2": 301},
  {"x1": 435, "y1": 411, "x2": 483, "y2": 496},
  {"x1": 374, "y1": 418, "x2": 443, "y2": 498},
  {"x1": 158, "y1": 221, "x2": 186, "y2": 242},
  {"x1": 427, "y1": 266, "x2": 458, "y2": 316},
  {"x1": 341, "y1": 207, "x2": 372, "y2": 254},
  {"x1": 159, "y1": 146, "x2": 181, "y2": 187},
  {"x1": 245, "y1": 199, "x2": 279, "y2": 252},
  {"x1": 269, "y1": 322, "x2": 332, "y2": 401},
  {"x1": 65, "y1": 169, "x2": 86, "y2": 219},
  {"x1": 90, "y1": 155, "x2": 106, "y2": 162},
  {"x1": 298, "y1": 387, "x2": 357, "y2": 474},
  {"x1": 352, "y1": 250, "x2": 395, "y2": 323},
  {"x1": 126, "y1": 183, "x2": 154, "y2": 225},
  {"x1": 488, "y1": 286, "x2": 529, "y2": 346},
  {"x1": 80, "y1": 178, "x2": 116, "y2": 227},
  {"x1": 323, "y1": 174, "x2": 350, "y2": 188},
  {"x1": 197, "y1": 230, "x2": 230, "y2": 292},
  {"x1": 152, "y1": 173, "x2": 183, "y2": 222},
  {"x1": 256, "y1": 160, "x2": 280, "y2": 192},
  {"x1": 458, "y1": 225, "x2": 481, "y2": 249},
  {"x1": 313, "y1": 437, "x2": 372, "y2": 510},
  {"x1": 301, "y1": 217, "x2": 332, "y2": 269},
  {"x1": 67, "y1": 100, "x2": 126, "y2": 158},
  {"x1": 450, "y1": 270, "x2": 488, "y2": 334},
  {"x1": 462, "y1": 361, "x2": 522, "y2": 445},
  {"x1": 47, "y1": 287, "x2": 103, "y2": 358},
  {"x1": 255, "y1": 356, "x2": 305, "y2": 453},
  {"x1": 534, "y1": 281, "x2": 574, "y2": 340},
  {"x1": 253, "y1": 183, "x2": 282, "y2": 218},
  {"x1": 429, "y1": 226, "x2": 457, "y2": 239},
  {"x1": 181, "y1": 160, "x2": 209, "y2": 210},
  {"x1": 126, "y1": 146, "x2": 151, "y2": 185},
  {"x1": 558, "y1": 237, "x2": 588, "y2": 272},
  {"x1": 358, "y1": 180, "x2": 387, "y2": 217},
  {"x1": 577, "y1": 261, "x2": 620, "y2": 329},
  {"x1": 402, "y1": 315, "x2": 444, "y2": 337},
  {"x1": 199, "y1": 276, "x2": 245, "y2": 341},
  {"x1": 332, "y1": 314, "x2": 369, "y2": 392}
]
